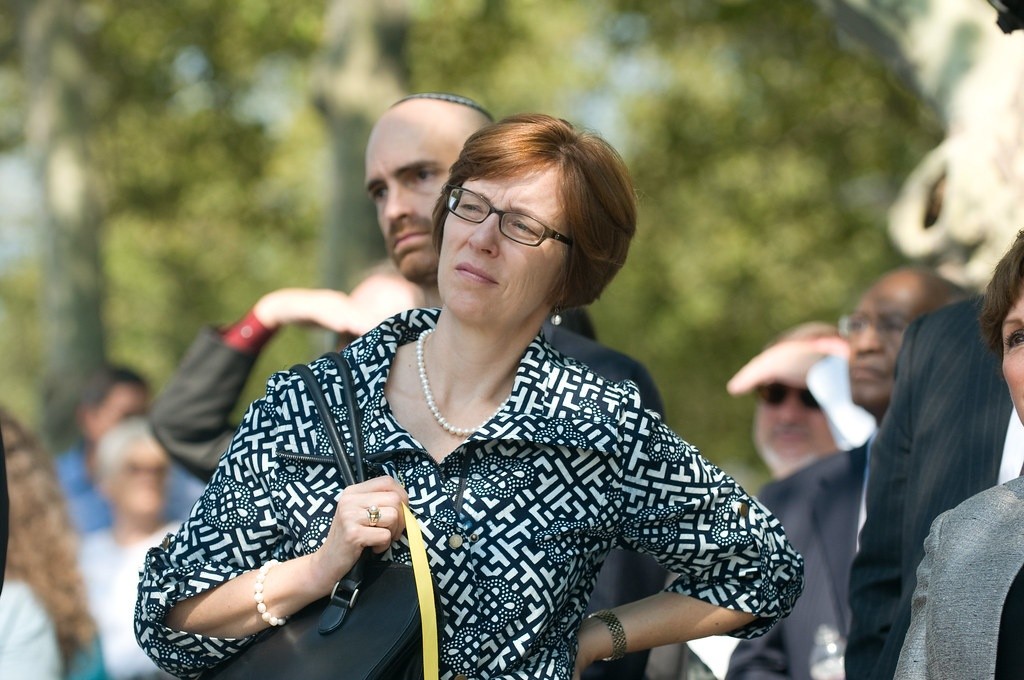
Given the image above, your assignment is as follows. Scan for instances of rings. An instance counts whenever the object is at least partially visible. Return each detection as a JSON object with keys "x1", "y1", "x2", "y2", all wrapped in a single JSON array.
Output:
[{"x1": 366, "y1": 505, "x2": 382, "y2": 527}]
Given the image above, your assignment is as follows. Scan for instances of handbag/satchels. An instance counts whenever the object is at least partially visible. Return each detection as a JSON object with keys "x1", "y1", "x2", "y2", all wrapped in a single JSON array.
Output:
[{"x1": 200, "y1": 554, "x2": 443, "y2": 680}]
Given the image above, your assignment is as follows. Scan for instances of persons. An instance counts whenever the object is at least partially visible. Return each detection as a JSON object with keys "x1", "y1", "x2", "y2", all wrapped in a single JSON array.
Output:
[
  {"x1": 891, "y1": 219, "x2": 1024, "y2": 680},
  {"x1": 144, "y1": 256, "x2": 428, "y2": 496},
  {"x1": 724, "y1": 265, "x2": 982, "y2": 680},
  {"x1": 679, "y1": 320, "x2": 879, "y2": 680},
  {"x1": 1, "y1": 360, "x2": 195, "y2": 680},
  {"x1": 840, "y1": 293, "x2": 1024, "y2": 680},
  {"x1": 130, "y1": 112, "x2": 806, "y2": 679},
  {"x1": 360, "y1": 92, "x2": 669, "y2": 680}
]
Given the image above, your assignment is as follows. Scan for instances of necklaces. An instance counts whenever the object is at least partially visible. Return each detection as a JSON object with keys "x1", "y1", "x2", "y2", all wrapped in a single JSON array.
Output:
[{"x1": 415, "y1": 326, "x2": 513, "y2": 439}]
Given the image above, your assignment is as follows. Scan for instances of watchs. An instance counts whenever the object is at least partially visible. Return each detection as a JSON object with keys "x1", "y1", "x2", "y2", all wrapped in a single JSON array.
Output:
[{"x1": 587, "y1": 608, "x2": 627, "y2": 662}]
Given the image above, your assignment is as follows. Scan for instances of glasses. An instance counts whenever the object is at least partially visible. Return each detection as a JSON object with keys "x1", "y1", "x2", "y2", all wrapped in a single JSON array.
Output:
[
  {"x1": 758, "y1": 384, "x2": 819, "y2": 408},
  {"x1": 838, "y1": 312, "x2": 911, "y2": 339},
  {"x1": 445, "y1": 184, "x2": 580, "y2": 247}
]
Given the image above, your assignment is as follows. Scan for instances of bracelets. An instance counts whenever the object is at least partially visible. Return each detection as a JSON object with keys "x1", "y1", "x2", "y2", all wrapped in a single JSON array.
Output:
[{"x1": 254, "y1": 558, "x2": 292, "y2": 627}]
